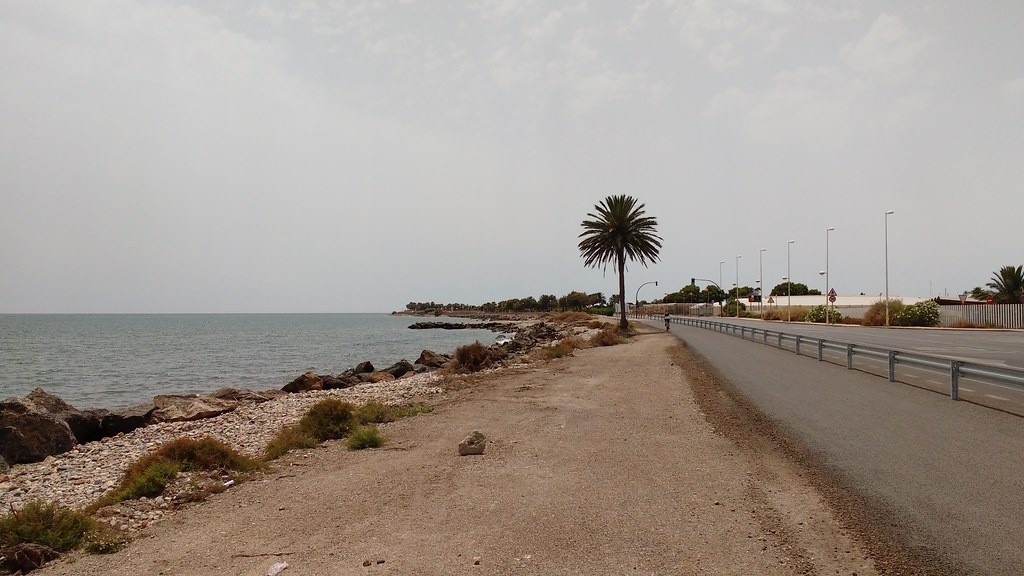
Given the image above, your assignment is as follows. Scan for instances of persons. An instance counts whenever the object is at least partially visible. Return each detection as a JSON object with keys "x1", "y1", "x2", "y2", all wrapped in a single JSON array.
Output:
[{"x1": 665, "y1": 311, "x2": 670, "y2": 329}]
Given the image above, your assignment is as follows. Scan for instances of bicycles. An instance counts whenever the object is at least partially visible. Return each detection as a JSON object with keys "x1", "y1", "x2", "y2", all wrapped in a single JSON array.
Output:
[{"x1": 665, "y1": 319, "x2": 670, "y2": 331}]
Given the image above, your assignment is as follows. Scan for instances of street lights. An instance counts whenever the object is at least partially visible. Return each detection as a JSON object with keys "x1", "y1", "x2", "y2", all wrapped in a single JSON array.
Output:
[
  {"x1": 819, "y1": 227, "x2": 834, "y2": 323},
  {"x1": 885, "y1": 211, "x2": 894, "y2": 325},
  {"x1": 756, "y1": 248, "x2": 766, "y2": 320},
  {"x1": 720, "y1": 261, "x2": 725, "y2": 289},
  {"x1": 782, "y1": 240, "x2": 795, "y2": 322},
  {"x1": 732, "y1": 255, "x2": 742, "y2": 318}
]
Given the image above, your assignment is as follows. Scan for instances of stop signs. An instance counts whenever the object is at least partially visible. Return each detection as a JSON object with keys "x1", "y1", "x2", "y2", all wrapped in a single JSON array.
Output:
[{"x1": 829, "y1": 296, "x2": 836, "y2": 302}]
